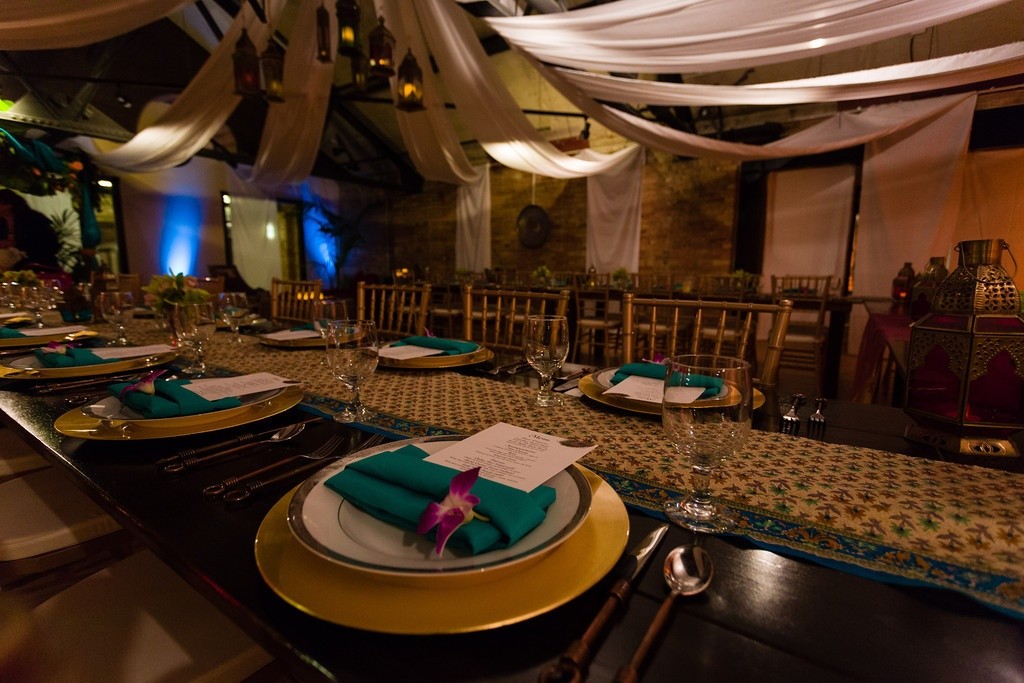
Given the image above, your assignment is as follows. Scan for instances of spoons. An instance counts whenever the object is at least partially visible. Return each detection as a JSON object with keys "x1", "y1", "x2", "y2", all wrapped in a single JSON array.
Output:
[
  {"x1": 612, "y1": 543, "x2": 713, "y2": 683},
  {"x1": 161, "y1": 422, "x2": 306, "y2": 475}
]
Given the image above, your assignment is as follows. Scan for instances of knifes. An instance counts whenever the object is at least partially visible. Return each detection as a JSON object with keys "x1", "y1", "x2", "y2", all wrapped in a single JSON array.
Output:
[
  {"x1": 553, "y1": 369, "x2": 598, "y2": 391},
  {"x1": 534, "y1": 523, "x2": 672, "y2": 683},
  {"x1": 155, "y1": 417, "x2": 326, "y2": 465}
]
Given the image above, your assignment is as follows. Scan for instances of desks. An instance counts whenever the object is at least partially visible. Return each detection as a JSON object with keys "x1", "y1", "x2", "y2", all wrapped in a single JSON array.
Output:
[
  {"x1": 0, "y1": 301, "x2": 1024, "y2": 683},
  {"x1": 866, "y1": 298, "x2": 1024, "y2": 473}
]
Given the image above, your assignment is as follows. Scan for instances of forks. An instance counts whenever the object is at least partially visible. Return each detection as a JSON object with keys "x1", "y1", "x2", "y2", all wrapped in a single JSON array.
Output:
[
  {"x1": 220, "y1": 431, "x2": 385, "y2": 502},
  {"x1": 810, "y1": 396, "x2": 828, "y2": 421},
  {"x1": 783, "y1": 393, "x2": 806, "y2": 423},
  {"x1": 203, "y1": 435, "x2": 344, "y2": 496}
]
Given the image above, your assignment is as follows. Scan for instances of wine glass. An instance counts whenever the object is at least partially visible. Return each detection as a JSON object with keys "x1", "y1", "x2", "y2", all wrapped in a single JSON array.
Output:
[
  {"x1": 173, "y1": 290, "x2": 247, "y2": 375},
  {"x1": 658, "y1": 353, "x2": 757, "y2": 532},
  {"x1": 522, "y1": 313, "x2": 568, "y2": 408},
  {"x1": 308, "y1": 298, "x2": 380, "y2": 423},
  {"x1": 0, "y1": 266, "x2": 144, "y2": 348}
]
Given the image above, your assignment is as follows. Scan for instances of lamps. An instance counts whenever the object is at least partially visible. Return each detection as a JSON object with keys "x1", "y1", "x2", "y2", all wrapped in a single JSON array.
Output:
[{"x1": 231, "y1": 0, "x2": 427, "y2": 113}]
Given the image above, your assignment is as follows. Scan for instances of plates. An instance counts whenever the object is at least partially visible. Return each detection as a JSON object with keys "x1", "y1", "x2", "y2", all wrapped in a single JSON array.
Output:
[
  {"x1": 576, "y1": 365, "x2": 764, "y2": 415},
  {"x1": 260, "y1": 321, "x2": 366, "y2": 348},
  {"x1": 53, "y1": 369, "x2": 307, "y2": 441},
  {"x1": 0, "y1": 323, "x2": 97, "y2": 349},
  {"x1": 212, "y1": 309, "x2": 267, "y2": 328},
  {"x1": 375, "y1": 335, "x2": 492, "y2": 369},
  {"x1": 0, "y1": 334, "x2": 183, "y2": 382},
  {"x1": 252, "y1": 433, "x2": 632, "y2": 638}
]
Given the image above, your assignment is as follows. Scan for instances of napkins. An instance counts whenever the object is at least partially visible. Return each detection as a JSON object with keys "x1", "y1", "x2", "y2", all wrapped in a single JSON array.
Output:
[
  {"x1": 0, "y1": 327, "x2": 28, "y2": 338},
  {"x1": 326, "y1": 444, "x2": 556, "y2": 552},
  {"x1": 390, "y1": 337, "x2": 479, "y2": 357},
  {"x1": 34, "y1": 349, "x2": 120, "y2": 368},
  {"x1": 109, "y1": 375, "x2": 242, "y2": 418},
  {"x1": 610, "y1": 363, "x2": 724, "y2": 400},
  {"x1": 291, "y1": 319, "x2": 355, "y2": 334}
]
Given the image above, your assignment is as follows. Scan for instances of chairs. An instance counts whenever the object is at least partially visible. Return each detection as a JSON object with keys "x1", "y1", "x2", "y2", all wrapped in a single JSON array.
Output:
[
  {"x1": 0, "y1": 426, "x2": 271, "y2": 683},
  {"x1": 268, "y1": 271, "x2": 831, "y2": 405}
]
